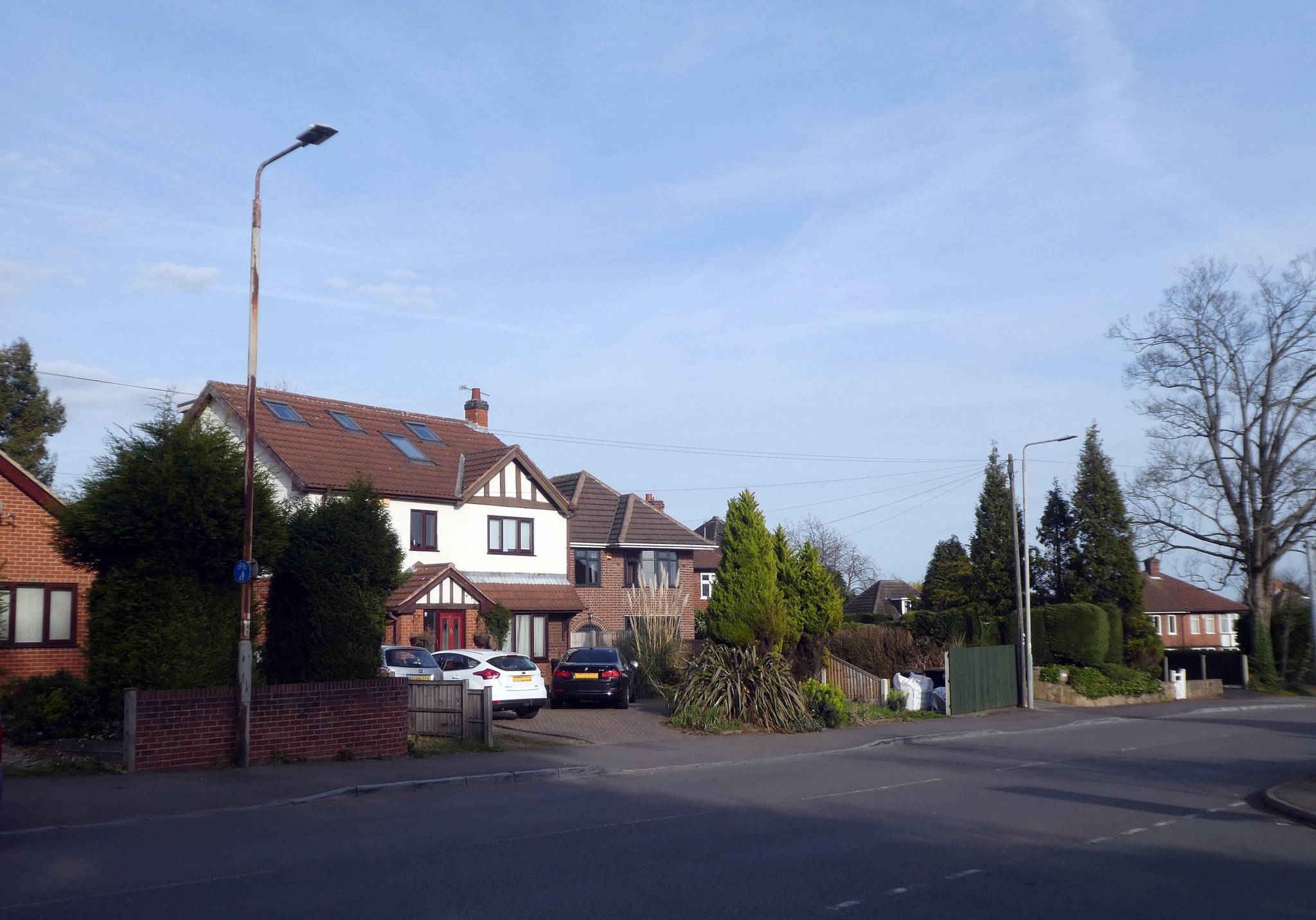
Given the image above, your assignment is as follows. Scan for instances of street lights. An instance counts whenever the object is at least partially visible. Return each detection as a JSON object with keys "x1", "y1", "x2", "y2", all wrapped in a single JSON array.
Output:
[
  {"x1": 236, "y1": 124, "x2": 340, "y2": 770},
  {"x1": 1020, "y1": 434, "x2": 1081, "y2": 710}
]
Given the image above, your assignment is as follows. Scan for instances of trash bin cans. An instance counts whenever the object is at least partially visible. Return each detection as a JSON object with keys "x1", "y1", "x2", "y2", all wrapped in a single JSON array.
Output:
[
  {"x1": 899, "y1": 670, "x2": 924, "y2": 678},
  {"x1": 923, "y1": 667, "x2": 945, "y2": 689}
]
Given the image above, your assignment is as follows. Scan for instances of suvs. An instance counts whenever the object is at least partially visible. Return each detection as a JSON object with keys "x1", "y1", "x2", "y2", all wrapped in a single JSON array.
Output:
[{"x1": 411, "y1": 648, "x2": 548, "y2": 719}]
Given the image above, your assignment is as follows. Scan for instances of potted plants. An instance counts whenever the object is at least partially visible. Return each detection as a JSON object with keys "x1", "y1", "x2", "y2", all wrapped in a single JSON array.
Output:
[
  {"x1": 409, "y1": 627, "x2": 438, "y2": 646},
  {"x1": 472, "y1": 630, "x2": 499, "y2": 650}
]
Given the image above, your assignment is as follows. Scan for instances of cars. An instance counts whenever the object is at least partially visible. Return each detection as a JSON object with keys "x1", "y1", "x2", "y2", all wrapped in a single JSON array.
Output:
[
  {"x1": 379, "y1": 644, "x2": 443, "y2": 680},
  {"x1": 550, "y1": 645, "x2": 640, "y2": 709}
]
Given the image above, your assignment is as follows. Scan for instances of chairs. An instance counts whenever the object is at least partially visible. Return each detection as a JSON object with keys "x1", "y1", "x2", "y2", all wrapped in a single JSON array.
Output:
[
  {"x1": 503, "y1": 660, "x2": 511, "y2": 667},
  {"x1": 453, "y1": 657, "x2": 462, "y2": 671},
  {"x1": 394, "y1": 658, "x2": 403, "y2": 665},
  {"x1": 406, "y1": 658, "x2": 418, "y2": 665}
]
